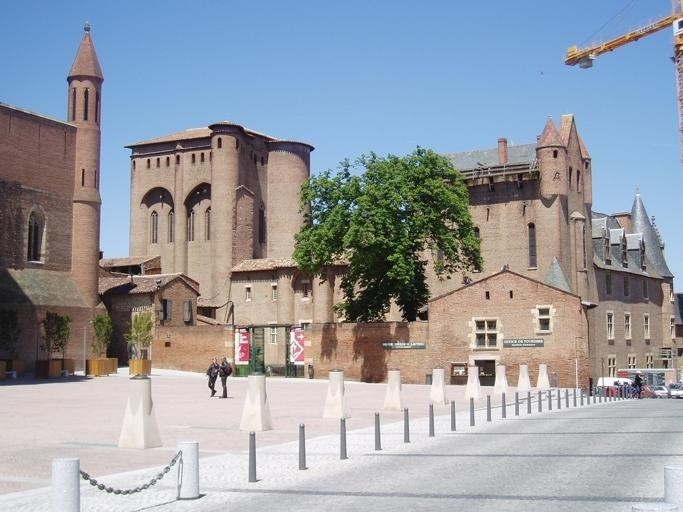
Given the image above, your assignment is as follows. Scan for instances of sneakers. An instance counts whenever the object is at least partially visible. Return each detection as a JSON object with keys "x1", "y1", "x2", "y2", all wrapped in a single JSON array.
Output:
[{"x1": 210, "y1": 390, "x2": 227, "y2": 398}]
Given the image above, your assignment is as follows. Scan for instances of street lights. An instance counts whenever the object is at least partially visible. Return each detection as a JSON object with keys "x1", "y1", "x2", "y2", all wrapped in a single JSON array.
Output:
[
  {"x1": 36, "y1": 319, "x2": 48, "y2": 379},
  {"x1": 84, "y1": 321, "x2": 95, "y2": 376}
]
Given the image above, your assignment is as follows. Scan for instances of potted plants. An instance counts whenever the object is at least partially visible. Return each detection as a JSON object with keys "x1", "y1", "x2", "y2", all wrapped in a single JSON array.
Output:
[{"x1": 0, "y1": 310, "x2": 153, "y2": 380}]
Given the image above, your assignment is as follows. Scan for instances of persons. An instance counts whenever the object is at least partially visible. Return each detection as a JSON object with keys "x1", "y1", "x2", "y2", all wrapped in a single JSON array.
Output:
[
  {"x1": 214, "y1": 357, "x2": 232, "y2": 398},
  {"x1": 204, "y1": 357, "x2": 219, "y2": 397},
  {"x1": 631, "y1": 371, "x2": 643, "y2": 399}
]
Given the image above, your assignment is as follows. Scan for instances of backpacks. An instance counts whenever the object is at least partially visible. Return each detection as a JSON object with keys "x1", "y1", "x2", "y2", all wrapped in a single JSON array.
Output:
[{"x1": 223, "y1": 363, "x2": 232, "y2": 376}]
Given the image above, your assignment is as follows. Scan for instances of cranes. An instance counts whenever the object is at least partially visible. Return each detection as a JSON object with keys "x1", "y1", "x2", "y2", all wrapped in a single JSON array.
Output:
[{"x1": 563, "y1": 0, "x2": 683, "y2": 166}]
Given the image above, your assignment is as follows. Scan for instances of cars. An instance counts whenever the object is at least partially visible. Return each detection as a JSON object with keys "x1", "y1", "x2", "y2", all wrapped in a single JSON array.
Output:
[{"x1": 596, "y1": 376, "x2": 683, "y2": 399}]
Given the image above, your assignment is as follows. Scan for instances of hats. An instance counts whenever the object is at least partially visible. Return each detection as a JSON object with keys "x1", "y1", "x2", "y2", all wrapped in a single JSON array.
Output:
[{"x1": 636, "y1": 371, "x2": 642, "y2": 374}]
